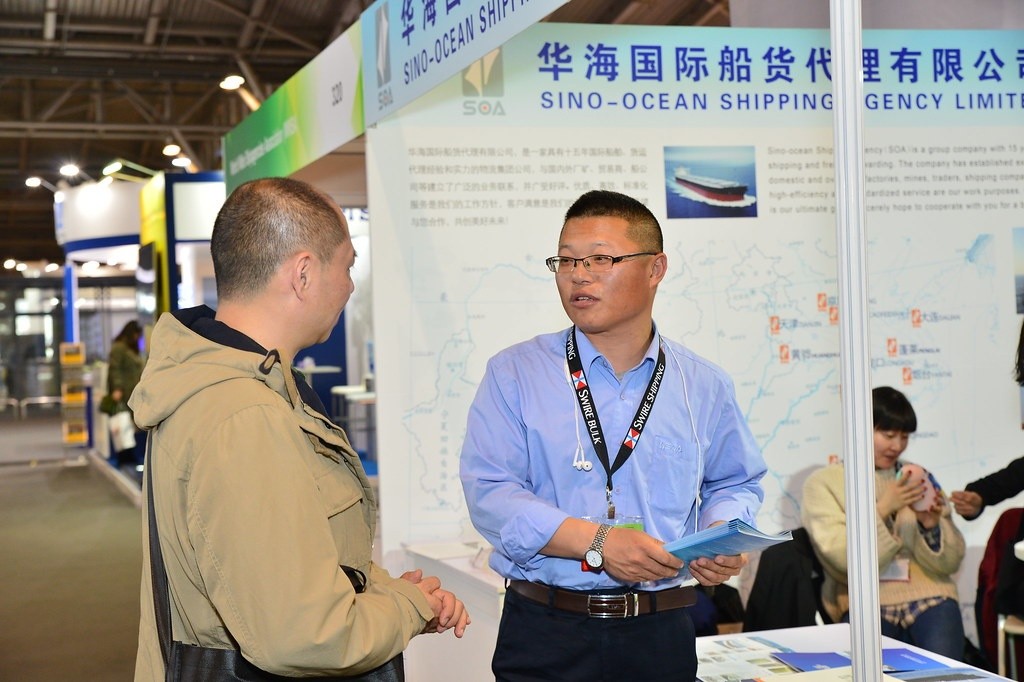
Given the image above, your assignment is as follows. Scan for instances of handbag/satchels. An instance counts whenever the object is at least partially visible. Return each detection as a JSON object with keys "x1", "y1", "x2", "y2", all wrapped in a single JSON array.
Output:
[
  {"x1": 161, "y1": 638, "x2": 407, "y2": 682},
  {"x1": 107, "y1": 411, "x2": 137, "y2": 453}
]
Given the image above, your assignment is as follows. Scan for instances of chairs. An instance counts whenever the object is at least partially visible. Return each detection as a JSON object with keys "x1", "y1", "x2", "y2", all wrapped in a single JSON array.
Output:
[
  {"x1": 763, "y1": 528, "x2": 833, "y2": 625},
  {"x1": 977, "y1": 507, "x2": 1024, "y2": 679}
]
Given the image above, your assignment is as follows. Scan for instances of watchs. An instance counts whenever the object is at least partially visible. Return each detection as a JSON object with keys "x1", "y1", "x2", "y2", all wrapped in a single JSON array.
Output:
[{"x1": 585, "y1": 524, "x2": 613, "y2": 569}]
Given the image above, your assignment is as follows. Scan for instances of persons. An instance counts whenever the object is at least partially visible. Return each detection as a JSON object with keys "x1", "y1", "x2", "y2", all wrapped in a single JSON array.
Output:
[
  {"x1": 98, "y1": 320, "x2": 147, "y2": 471},
  {"x1": 127, "y1": 177, "x2": 471, "y2": 682},
  {"x1": 460, "y1": 188, "x2": 768, "y2": 682},
  {"x1": 802, "y1": 386, "x2": 966, "y2": 664},
  {"x1": 951, "y1": 318, "x2": 1024, "y2": 623}
]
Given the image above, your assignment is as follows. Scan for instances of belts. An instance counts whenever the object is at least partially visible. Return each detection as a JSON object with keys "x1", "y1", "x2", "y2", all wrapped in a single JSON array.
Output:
[{"x1": 508, "y1": 579, "x2": 698, "y2": 620}]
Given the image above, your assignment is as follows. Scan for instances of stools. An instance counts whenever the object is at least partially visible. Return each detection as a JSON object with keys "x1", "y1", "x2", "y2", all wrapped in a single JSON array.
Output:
[
  {"x1": 344, "y1": 392, "x2": 377, "y2": 475},
  {"x1": 329, "y1": 385, "x2": 366, "y2": 429}
]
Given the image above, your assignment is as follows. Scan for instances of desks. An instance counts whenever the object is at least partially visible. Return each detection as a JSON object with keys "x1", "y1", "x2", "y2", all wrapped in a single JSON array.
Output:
[
  {"x1": 293, "y1": 365, "x2": 340, "y2": 393},
  {"x1": 698, "y1": 623, "x2": 1018, "y2": 682},
  {"x1": 1015, "y1": 539, "x2": 1024, "y2": 562}
]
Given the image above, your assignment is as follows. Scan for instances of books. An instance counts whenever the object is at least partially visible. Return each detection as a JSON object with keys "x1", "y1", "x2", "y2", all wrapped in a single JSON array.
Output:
[{"x1": 650, "y1": 518, "x2": 794, "y2": 577}]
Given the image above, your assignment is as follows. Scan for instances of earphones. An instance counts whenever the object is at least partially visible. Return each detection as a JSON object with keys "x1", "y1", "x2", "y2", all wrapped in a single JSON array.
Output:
[
  {"x1": 573, "y1": 448, "x2": 583, "y2": 471},
  {"x1": 581, "y1": 450, "x2": 593, "y2": 470}
]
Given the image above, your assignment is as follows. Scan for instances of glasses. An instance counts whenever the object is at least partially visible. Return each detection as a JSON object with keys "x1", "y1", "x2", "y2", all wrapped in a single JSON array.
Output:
[{"x1": 545, "y1": 252, "x2": 658, "y2": 274}]
